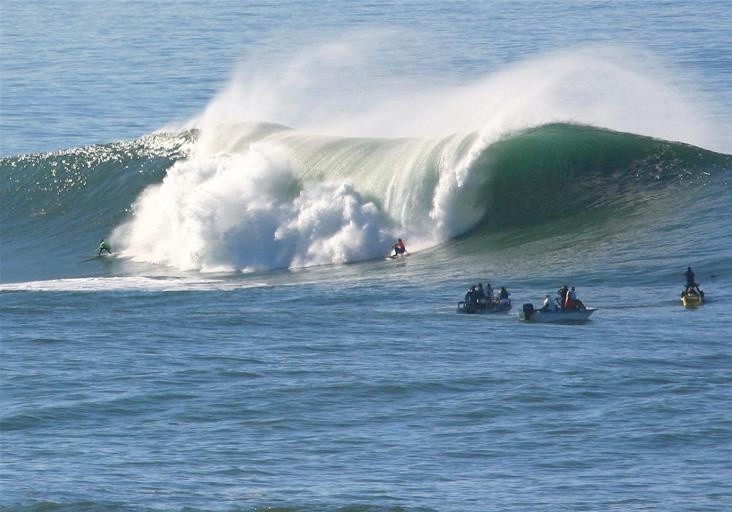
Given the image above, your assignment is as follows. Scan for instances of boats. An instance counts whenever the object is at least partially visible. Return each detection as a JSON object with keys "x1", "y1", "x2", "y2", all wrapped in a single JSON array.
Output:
[
  {"x1": 681, "y1": 283, "x2": 705, "y2": 308},
  {"x1": 522, "y1": 303, "x2": 599, "y2": 322},
  {"x1": 457, "y1": 290, "x2": 512, "y2": 314}
]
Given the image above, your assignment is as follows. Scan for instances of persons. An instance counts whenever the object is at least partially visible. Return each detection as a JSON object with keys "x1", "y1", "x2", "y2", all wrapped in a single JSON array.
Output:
[
  {"x1": 684, "y1": 267, "x2": 695, "y2": 294},
  {"x1": 98, "y1": 240, "x2": 112, "y2": 255},
  {"x1": 464, "y1": 283, "x2": 509, "y2": 307},
  {"x1": 539, "y1": 285, "x2": 585, "y2": 311},
  {"x1": 392, "y1": 238, "x2": 407, "y2": 256}
]
